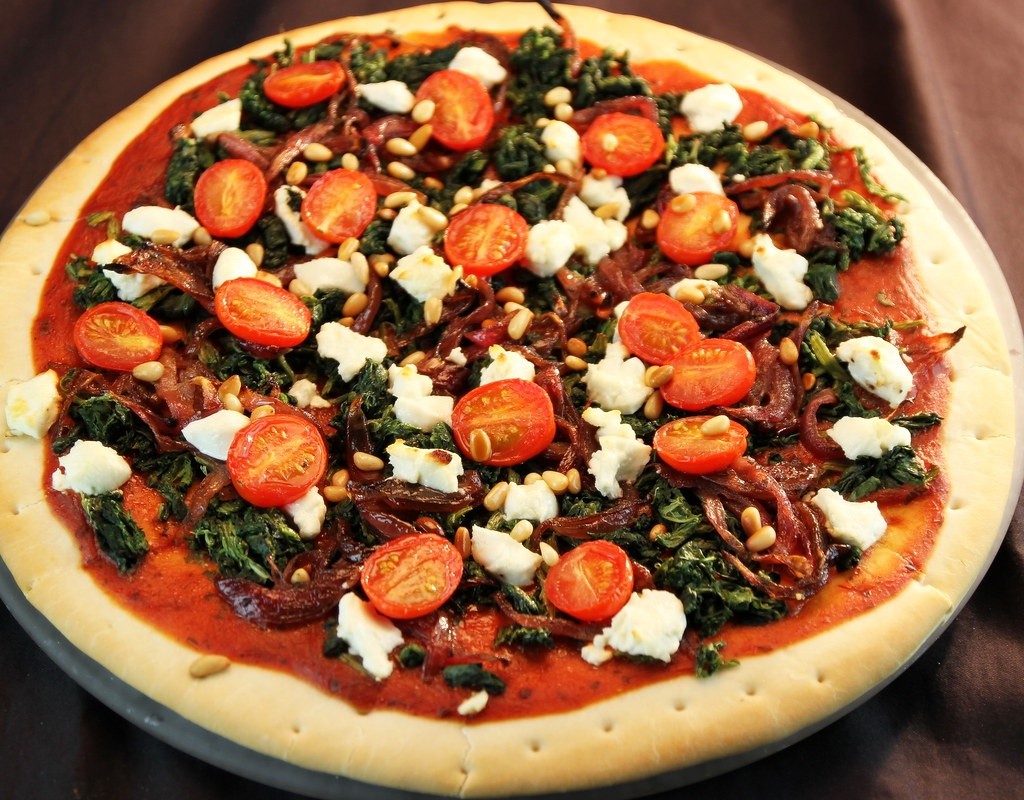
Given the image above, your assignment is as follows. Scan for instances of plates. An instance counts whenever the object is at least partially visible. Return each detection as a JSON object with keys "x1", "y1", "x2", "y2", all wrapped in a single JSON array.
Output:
[{"x1": 0, "y1": 42, "x2": 1024, "y2": 800}]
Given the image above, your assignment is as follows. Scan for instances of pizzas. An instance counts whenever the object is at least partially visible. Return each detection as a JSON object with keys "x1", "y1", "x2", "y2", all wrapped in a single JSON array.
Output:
[{"x1": 0, "y1": 0, "x2": 1024, "y2": 800}]
